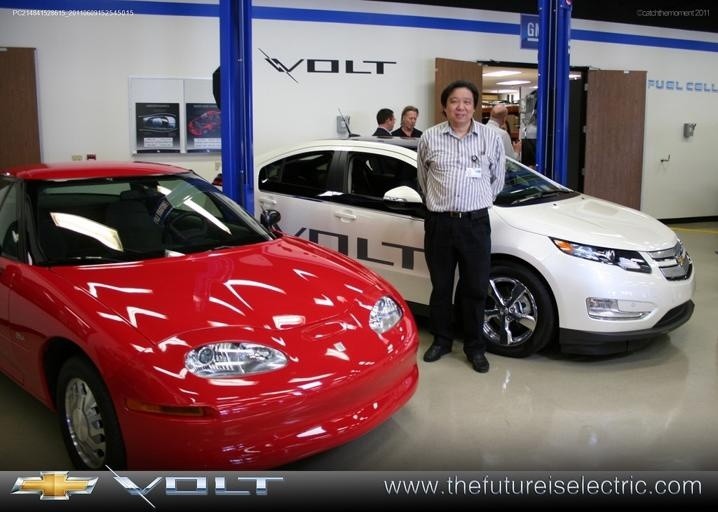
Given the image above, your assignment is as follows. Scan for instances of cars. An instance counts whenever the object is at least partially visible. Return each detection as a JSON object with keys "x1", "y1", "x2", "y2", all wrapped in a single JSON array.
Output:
[
  {"x1": 209, "y1": 108, "x2": 695, "y2": 360},
  {"x1": 188, "y1": 109, "x2": 221, "y2": 136},
  {"x1": 137, "y1": 112, "x2": 178, "y2": 138},
  {"x1": 0, "y1": 161, "x2": 418, "y2": 472}
]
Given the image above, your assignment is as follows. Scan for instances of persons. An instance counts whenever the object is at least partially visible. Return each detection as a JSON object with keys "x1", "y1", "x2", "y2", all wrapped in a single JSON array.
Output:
[
  {"x1": 486, "y1": 103, "x2": 522, "y2": 173},
  {"x1": 369, "y1": 109, "x2": 395, "y2": 173},
  {"x1": 417, "y1": 80, "x2": 506, "y2": 374},
  {"x1": 391, "y1": 106, "x2": 423, "y2": 180}
]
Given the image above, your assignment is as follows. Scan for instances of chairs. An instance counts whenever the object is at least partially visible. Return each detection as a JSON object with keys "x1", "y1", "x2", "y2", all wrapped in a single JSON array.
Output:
[
  {"x1": 1, "y1": 208, "x2": 76, "y2": 264},
  {"x1": 95, "y1": 199, "x2": 163, "y2": 256}
]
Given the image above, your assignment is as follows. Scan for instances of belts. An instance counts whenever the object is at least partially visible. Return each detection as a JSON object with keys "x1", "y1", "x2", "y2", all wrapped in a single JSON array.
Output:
[{"x1": 427, "y1": 208, "x2": 489, "y2": 219}]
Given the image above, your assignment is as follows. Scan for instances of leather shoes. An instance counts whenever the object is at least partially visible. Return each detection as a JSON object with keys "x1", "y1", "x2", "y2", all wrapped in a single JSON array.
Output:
[
  {"x1": 466, "y1": 348, "x2": 489, "y2": 373},
  {"x1": 423, "y1": 340, "x2": 452, "y2": 362}
]
